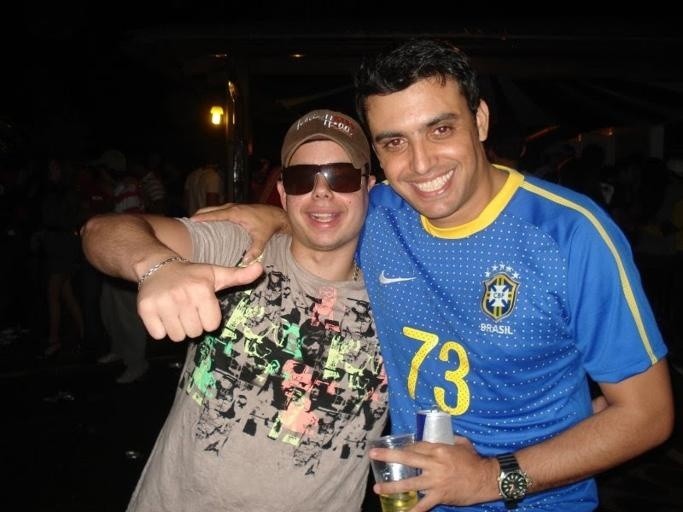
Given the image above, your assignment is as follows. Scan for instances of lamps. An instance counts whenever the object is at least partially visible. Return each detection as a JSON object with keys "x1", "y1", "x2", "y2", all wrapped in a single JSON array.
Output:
[{"x1": 210, "y1": 106, "x2": 224, "y2": 126}]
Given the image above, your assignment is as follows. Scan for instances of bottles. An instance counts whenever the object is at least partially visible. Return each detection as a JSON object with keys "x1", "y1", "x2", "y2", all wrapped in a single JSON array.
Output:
[{"x1": 413, "y1": 408, "x2": 454, "y2": 495}]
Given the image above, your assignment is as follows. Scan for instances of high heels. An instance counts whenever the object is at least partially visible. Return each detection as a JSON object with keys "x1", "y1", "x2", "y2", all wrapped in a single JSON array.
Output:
[{"x1": 35, "y1": 341, "x2": 64, "y2": 360}]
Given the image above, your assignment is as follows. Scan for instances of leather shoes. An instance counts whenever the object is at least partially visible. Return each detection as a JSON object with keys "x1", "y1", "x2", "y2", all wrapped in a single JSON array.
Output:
[
  {"x1": 116, "y1": 360, "x2": 151, "y2": 384},
  {"x1": 96, "y1": 347, "x2": 121, "y2": 364}
]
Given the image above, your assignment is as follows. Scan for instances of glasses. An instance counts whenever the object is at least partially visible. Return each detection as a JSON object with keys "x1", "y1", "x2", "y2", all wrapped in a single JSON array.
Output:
[{"x1": 278, "y1": 163, "x2": 370, "y2": 195}]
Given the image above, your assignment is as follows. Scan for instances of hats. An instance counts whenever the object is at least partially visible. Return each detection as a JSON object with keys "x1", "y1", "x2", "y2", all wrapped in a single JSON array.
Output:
[{"x1": 280, "y1": 109, "x2": 372, "y2": 175}]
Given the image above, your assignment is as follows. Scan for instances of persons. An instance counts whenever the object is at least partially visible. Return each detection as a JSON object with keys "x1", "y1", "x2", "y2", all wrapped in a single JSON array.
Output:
[{"x1": 34, "y1": 35, "x2": 675, "y2": 512}]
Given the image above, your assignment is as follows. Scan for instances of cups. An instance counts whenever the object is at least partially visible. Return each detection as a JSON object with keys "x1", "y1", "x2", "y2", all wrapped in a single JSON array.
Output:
[{"x1": 368, "y1": 434, "x2": 423, "y2": 512}]
